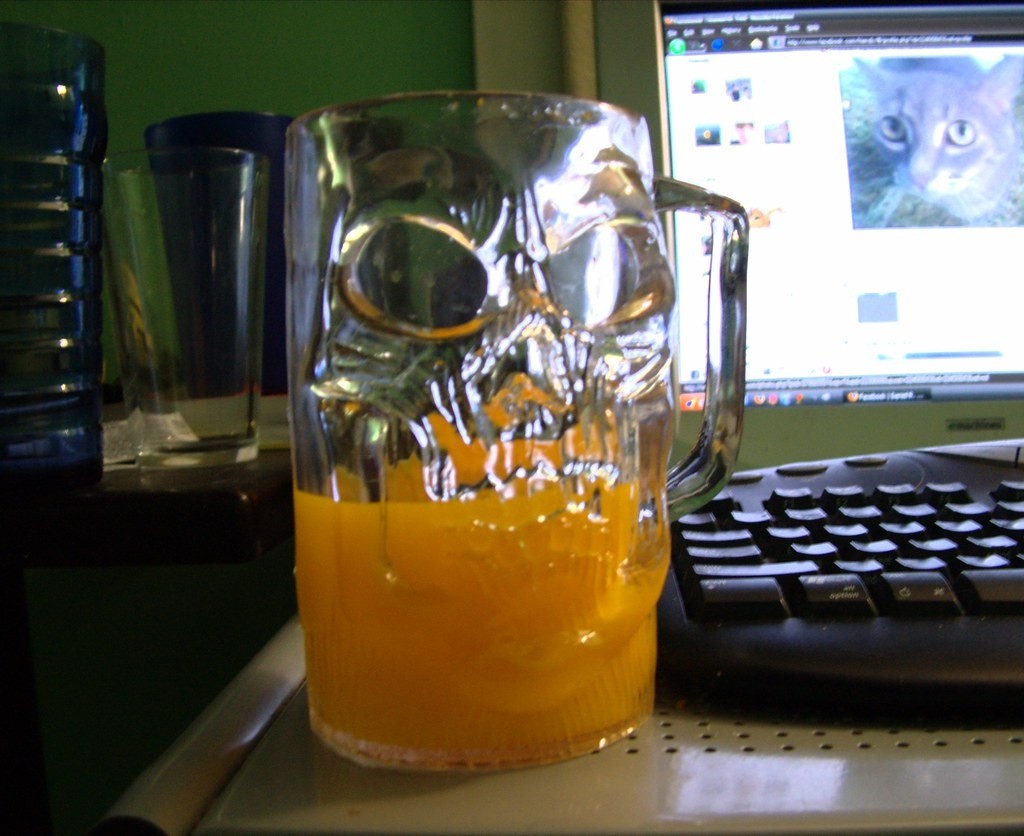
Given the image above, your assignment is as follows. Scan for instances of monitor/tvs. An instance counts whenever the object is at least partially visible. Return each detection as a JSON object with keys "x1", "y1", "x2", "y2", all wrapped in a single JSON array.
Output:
[{"x1": 654, "y1": 0, "x2": 1024, "y2": 467}]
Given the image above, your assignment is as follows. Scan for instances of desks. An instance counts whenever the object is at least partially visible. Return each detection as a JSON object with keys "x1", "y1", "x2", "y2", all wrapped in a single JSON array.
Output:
[
  {"x1": 0, "y1": 405, "x2": 301, "y2": 835},
  {"x1": 85, "y1": 615, "x2": 1024, "y2": 836}
]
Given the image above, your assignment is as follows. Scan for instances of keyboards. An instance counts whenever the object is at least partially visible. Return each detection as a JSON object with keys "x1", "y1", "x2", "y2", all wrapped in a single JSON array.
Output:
[{"x1": 656, "y1": 436, "x2": 1024, "y2": 711}]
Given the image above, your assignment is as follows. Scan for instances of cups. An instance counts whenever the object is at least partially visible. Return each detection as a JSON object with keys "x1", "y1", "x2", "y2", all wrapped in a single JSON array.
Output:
[
  {"x1": 285, "y1": 90, "x2": 749, "y2": 771},
  {"x1": 99, "y1": 147, "x2": 267, "y2": 471}
]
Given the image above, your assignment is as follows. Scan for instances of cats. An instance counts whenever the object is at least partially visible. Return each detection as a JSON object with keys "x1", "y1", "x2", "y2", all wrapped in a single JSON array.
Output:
[{"x1": 853, "y1": 54, "x2": 1024, "y2": 227}]
